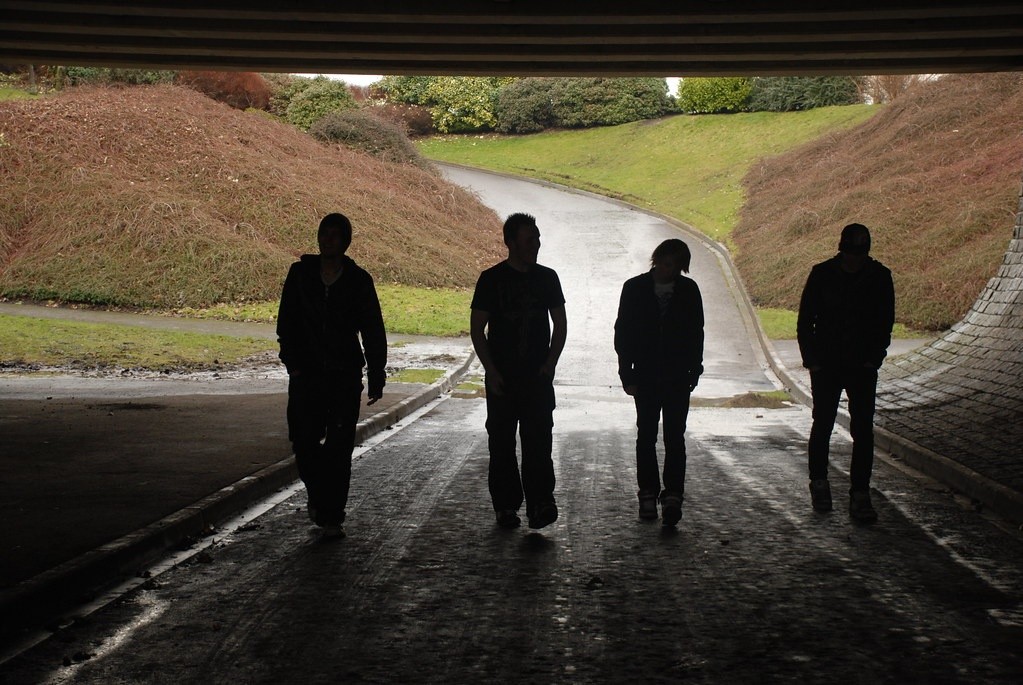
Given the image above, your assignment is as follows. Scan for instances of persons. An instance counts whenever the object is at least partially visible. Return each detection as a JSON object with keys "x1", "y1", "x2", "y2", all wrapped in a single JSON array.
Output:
[
  {"x1": 797, "y1": 223, "x2": 896, "y2": 516},
  {"x1": 609, "y1": 239, "x2": 708, "y2": 526},
  {"x1": 465, "y1": 212, "x2": 567, "y2": 540},
  {"x1": 275, "y1": 213, "x2": 389, "y2": 537}
]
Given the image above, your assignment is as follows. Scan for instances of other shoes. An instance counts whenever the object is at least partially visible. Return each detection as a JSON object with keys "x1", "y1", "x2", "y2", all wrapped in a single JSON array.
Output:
[
  {"x1": 661, "y1": 501, "x2": 682, "y2": 526},
  {"x1": 497, "y1": 510, "x2": 521, "y2": 527},
  {"x1": 529, "y1": 504, "x2": 559, "y2": 528},
  {"x1": 850, "y1": 498, "x2": 878, "y2": 519},
  {"x1": 306, "y1": 489, "x2": 319, "y2": 513},
  {"x1": 640, "y1": 495, "x2": 658, "y2": 519},
  {"x1": 810, "y1": 481, "x2": 832, "y2": 510},
  {"x1": 315, "y1": 511, "x2": 341, "y2": 528}
]
